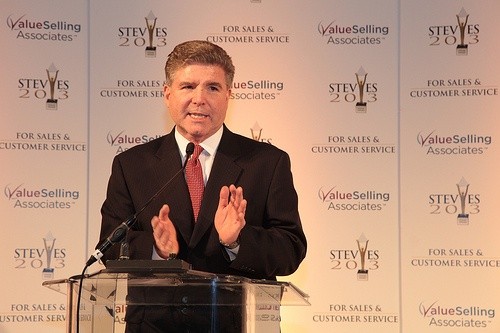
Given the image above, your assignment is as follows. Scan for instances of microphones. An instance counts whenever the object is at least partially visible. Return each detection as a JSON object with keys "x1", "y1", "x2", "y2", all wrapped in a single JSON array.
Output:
[{"x1": 86, "y1": 143, "x2": 194, "y2": 268}]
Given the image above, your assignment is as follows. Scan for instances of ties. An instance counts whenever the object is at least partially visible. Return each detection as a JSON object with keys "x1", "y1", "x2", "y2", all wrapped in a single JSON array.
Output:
[{"x1": 185, "y1": 144, "x2": 204, "y2": 221}]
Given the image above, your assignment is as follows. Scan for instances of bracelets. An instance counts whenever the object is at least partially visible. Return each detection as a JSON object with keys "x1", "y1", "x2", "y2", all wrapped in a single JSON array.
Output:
[{"x1": 219, "y1": 239, "x2": 239, "y2": 249}]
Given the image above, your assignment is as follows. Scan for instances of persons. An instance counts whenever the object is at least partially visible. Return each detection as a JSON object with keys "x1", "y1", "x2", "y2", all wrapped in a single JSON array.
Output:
[{"x1": 95, "y1": 42, "x2": 307, "y2": 333}]
